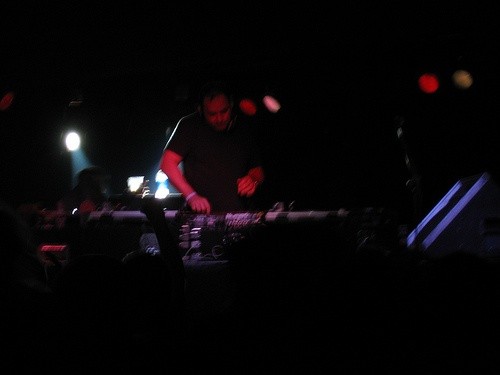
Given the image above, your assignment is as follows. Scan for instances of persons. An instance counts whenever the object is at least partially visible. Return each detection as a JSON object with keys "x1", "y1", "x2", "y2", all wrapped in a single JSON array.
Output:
[
  {"x1": 383, "y1": 86, "x2": 472, "y2": 227},
  {"x1": 158, "y1": 78, "x2": 276, "y2": 214}
]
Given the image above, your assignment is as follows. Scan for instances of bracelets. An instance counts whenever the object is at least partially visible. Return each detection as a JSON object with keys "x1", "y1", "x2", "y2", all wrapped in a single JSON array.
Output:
[
  {"x1": 246, "y1": 174, "x2": 258, "y2": 186},
  {"x1": 185, "y1": 191, "x2": 197, "y2": 202}
]
